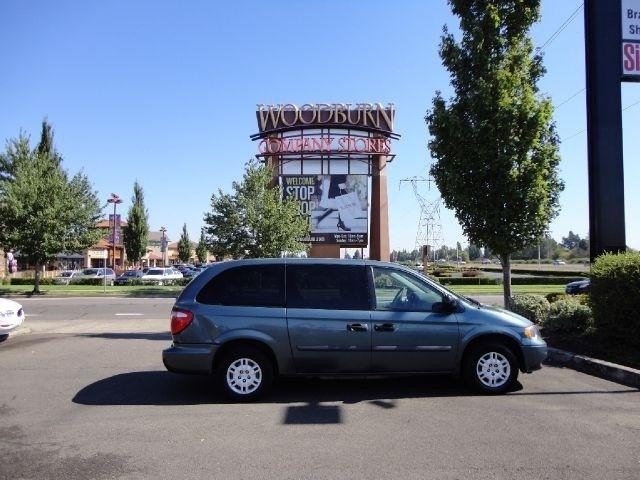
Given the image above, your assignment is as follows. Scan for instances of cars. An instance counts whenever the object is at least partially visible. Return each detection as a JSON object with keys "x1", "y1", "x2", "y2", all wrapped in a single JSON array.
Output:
[
  {"x1": 174, "y1": 263, "x2": 202, "y2": 280},
  {"x1": 141, "y1": 267, "x2": 183, "y2": 285},
  {"x1": 554, "y1": 260, "x2": 565, "y2": 265},
  {"x1": 454, "y1": 259, "x2": 466, "y2": 265},
  {"x1": 162, "y1": 257, "x2": 547, "y2": 399},
  {"x1": 60, "y1": 266, "x2": 144, "y2": 285},
  {"x1": 0, "y1": 297, "x2": 25, "y2": 338},
  {"x1": 481, "y1": 258, "x2": 491, "y2": 265},
  {"x1": 565, "y1": 278, "x2": 590, "y2": 294},
  {"x1": 439, "y1": 258, "x2": 447, "y2": 263}
]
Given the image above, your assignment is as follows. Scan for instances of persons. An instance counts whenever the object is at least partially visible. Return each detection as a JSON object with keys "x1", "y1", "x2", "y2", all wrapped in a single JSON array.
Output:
[
  {"x1": 316, "y1": 175, "x2": 351, "y2": 231},
  {"x1": 8, "y1": 257, "x2": 17, "y2": 277}
]
